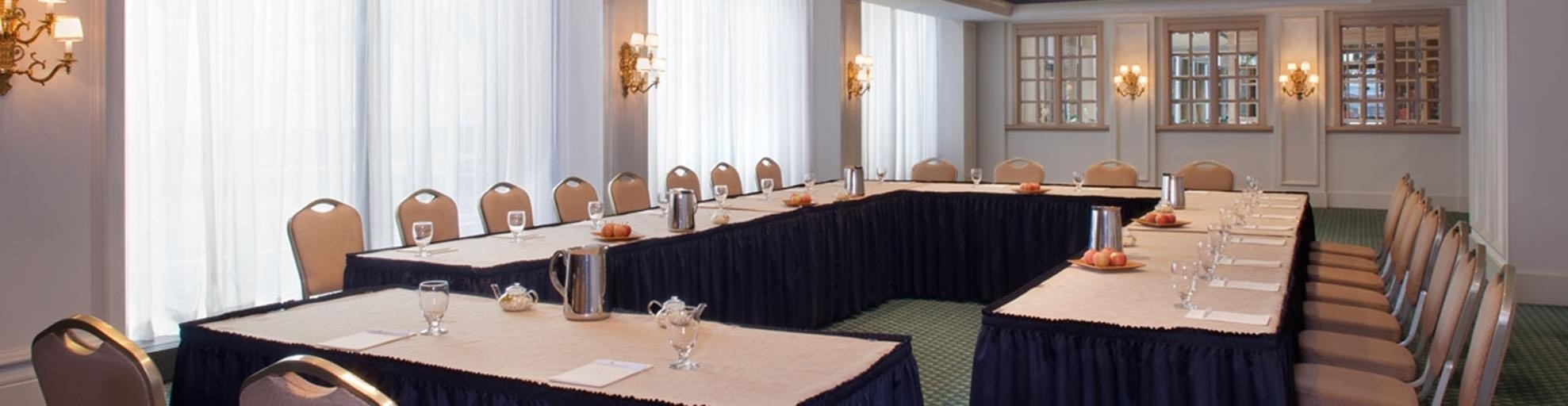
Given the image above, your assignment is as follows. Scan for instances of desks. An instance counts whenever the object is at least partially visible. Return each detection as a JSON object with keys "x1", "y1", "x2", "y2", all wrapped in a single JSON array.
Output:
[
  {"x1": 337, "y1": 180, "x2": 898, "y2": 331},
  {"x1": 971, "y1": 188, "x2": 1316, "y2": 406},
  {"x1": 892, "y1": 178, "x2": 1164, "y2": 307},
  {"x1": 167, "y1": 285, "x2": 927, "y2": 405}
]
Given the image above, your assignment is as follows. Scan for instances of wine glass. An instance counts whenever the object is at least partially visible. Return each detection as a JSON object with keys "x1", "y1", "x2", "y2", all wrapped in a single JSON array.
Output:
[
  {"x1": 761, "y1": 178, "x2": 774, "y2": 202},
  {"x1": 1072, "y1": 170, "x2": 1084, "y2": 190},
  {"x1": 663, "y1": 306, "x2": 701, "y2": 369},
  {"x1": 656, "y1": 191, "x2": 669, "y2": 218},
  {"x1": 713, "y1": 185, "x2": 728, "y2": 211},
  {"x1": 412, "y1": 222, "x2": 433, "y2": 257},
  {"x1": 587, "y1": 201, "x2": 605, "y2": 230},
  {"x1": 1171, "y1": 175, "x2": 1260, "y2": 310},
  {"x1": 804, "y1": 174, "x2": 814, "y2": 194},
  {"x1": 970, "y1": 168, "x2": 983, "y2": 188},
  {"x1": 418, "y1": 280, "x2": 450, "y2": 337},
  {"x1": 876, "y1": 163, "x2": 888, "y2": 185},
  {"x1": 507, "y1": 211, "x2": 526, "y2": 243}
]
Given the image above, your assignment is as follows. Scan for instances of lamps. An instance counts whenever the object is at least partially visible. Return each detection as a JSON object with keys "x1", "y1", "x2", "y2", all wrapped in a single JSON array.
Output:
[
  {"x1": 1278, "y1": 62, "x2": 1319, "y2": 100},
  {"x1": 848, "y1": 55, "x2": 875, "y2": 101},
  {"x1": 1113, "y1": 65, "x2": 1148, "y2": 100},
  {"x1": 0, "y1": 0, "x2": 87, "y2": 99},
  {"x1": 619, "y1": 31, "x2": 669, "y2": 97}
]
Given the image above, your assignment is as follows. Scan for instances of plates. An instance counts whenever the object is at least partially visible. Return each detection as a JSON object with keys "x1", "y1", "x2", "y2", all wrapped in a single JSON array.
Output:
[
  {"x1": 591, "y1": 231, "x2": 643, "y2": 241},
  {"x1": 1066, "y1": 259, "x2": 1146, "y2": 273},
  {"x1": 1131, "y1": 218, "x2": 1191, "y2": 229},
  {"x1": 1011, "y1": 187, "x2": 1050, "y2": 195},
  {"x1": 782, "y1": 199, "x2": 818, "y2": 207}
]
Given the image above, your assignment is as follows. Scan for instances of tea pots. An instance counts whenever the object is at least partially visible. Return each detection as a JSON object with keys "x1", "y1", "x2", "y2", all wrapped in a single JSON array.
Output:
[
  {"x1": 668, "y1": 188, "x2": 698, "y2": 232},
  {"x1": 548, "y1": 244, "x2": 612, "y2": 321},
  {"x1": 648, "y1": 298, "x2": 707, "y2": 329},
  {"x1": 491, "y1": 283, "x2": 538, "y2": 312},
  {"x1": 1157, "y1": 172, "x2": 1185, "y2": 210},
  {"x1": 1088, "y1": 206, "x2": 1122, "y2": 255},
  {"x1": 844, "y1": 165, "x2": 864, "y2": 196}
]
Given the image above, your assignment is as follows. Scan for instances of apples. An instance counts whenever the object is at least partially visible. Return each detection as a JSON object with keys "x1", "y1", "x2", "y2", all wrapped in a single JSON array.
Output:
[
  {"x1": 1084, "y1": 248, "x2": 1126, "y2": 267},
  {"x1": 782, "y1": 191, "x2": 812, "y2": 205},
  {"x1": 600, "y1": 220, "x2": 632, "y2": 237},
  {"x1": 1020, "y1": 182, "x2": 1041, "y2": 191},
  {"x1": 1145, "y1": 212, "x2": 1176, "y2": 224}
]
